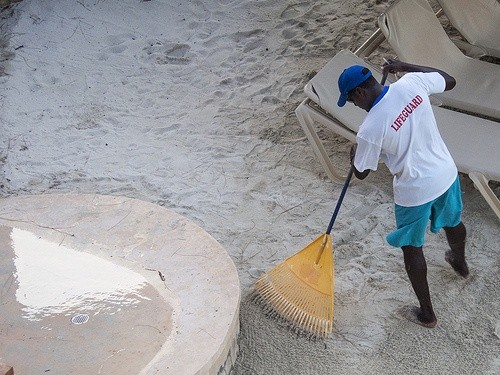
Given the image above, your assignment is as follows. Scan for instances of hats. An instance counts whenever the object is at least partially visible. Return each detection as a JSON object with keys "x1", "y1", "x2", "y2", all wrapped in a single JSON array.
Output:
[{"x1": 337, "y1": 65, "x2": 372, "y2": 107}]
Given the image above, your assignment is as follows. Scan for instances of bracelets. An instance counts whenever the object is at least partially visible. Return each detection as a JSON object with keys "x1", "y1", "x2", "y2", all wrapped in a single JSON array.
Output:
[{"x1": 350, "y1": 154, "x2": 355, "y2": 163}]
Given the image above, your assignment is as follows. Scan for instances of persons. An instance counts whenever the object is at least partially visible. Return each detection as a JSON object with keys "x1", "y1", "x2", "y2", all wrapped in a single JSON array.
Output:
[{"x1": 338, "y1": 58, "x2": 470, "y2": 327}]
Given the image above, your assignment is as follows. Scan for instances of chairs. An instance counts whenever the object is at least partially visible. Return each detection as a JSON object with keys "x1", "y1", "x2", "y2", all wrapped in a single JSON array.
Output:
[
  {"x1": 437, "y1": 0, "x2": 500, "y2": 58},
  {"x1": 296, "y1": 50, "x2": 500, "y2": 220},
  {"x1": 354, "y1": 0, "x2": 500, "y2": 120}
]
